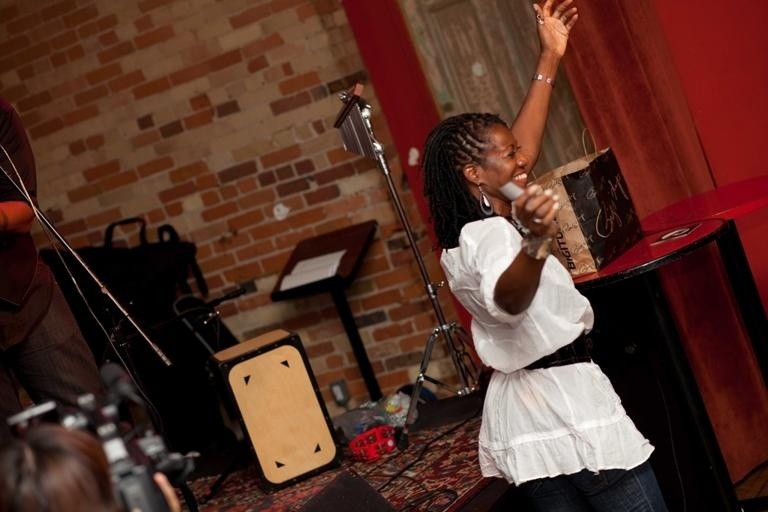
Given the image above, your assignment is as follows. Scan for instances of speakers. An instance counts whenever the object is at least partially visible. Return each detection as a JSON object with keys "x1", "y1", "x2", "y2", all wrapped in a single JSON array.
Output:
[{"x1": 294, "y1": 467, "x2": 402, "y2": 511}]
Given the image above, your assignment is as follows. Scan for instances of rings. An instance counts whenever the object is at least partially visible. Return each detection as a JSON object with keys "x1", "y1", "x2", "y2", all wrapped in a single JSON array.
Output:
[
  {"x1": 535, "y1": 14, "x2": 544, "y2": 24},
  {"x1": 532, "y1": 218, "x2": 543, "y2": 224}
]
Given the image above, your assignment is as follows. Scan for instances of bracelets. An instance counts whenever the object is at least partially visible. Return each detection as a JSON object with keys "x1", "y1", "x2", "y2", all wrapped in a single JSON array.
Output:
[
  {"x1": 528, "y1": 72, "x2": 557, "y2": 88},
  {"x1": 517, "y1": 235, "x2": 549, "y2": 263}
]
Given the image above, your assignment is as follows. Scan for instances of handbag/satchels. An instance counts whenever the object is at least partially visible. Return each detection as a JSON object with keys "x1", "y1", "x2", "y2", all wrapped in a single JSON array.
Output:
[{"x1": 527, "y1": 130, "x2": 644, "y2": 278}]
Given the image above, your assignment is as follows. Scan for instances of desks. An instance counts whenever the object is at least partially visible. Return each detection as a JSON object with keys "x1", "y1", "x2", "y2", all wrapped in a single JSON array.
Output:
[{"x1": 574, "y1": 217, "x2": 768, "y2": 512}]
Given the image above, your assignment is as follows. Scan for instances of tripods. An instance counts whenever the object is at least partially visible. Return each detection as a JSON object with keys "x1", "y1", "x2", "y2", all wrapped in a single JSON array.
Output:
[{"x1": 377, "y1": 161, "x2": 486, "y2": 453}]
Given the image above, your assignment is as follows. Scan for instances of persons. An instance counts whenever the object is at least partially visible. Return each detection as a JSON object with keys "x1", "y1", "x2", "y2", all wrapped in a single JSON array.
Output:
[
  {"x1": 420, "y1": 0, "x2": 671, "y2": 511},
  {"x1": 0, "y1": 422, "x2": 181, "y2": 511}
]
